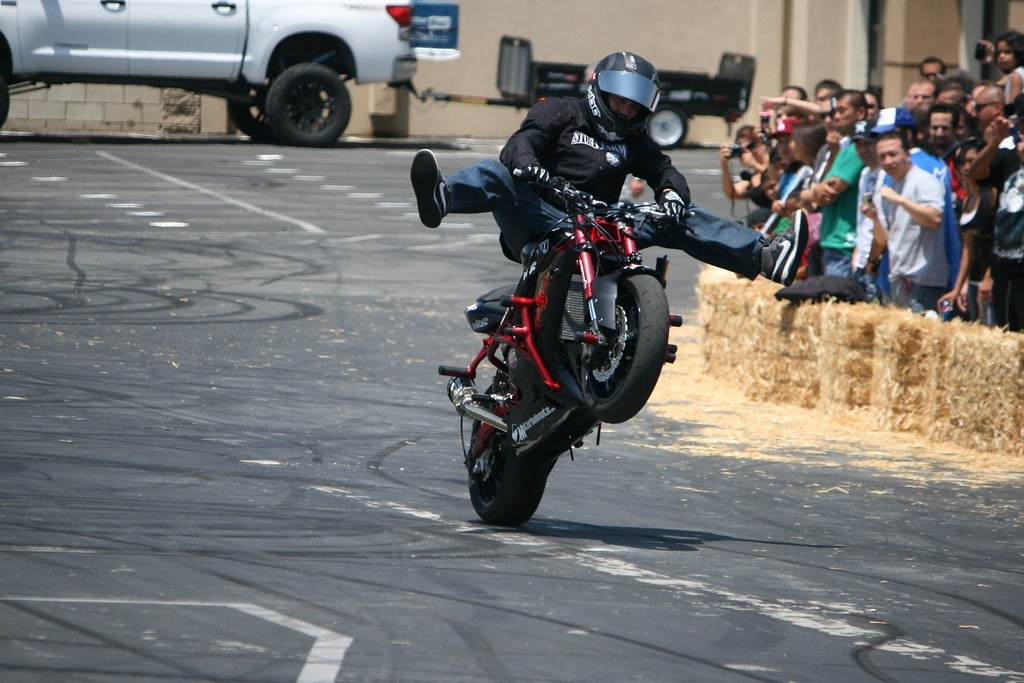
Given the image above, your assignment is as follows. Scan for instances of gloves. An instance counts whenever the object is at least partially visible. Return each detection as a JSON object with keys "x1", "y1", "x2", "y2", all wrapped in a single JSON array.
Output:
[
  {"x1": 524, "y1": 166, "x2": 550, "y2": 183},
  {"x1": 659, "y1": 189, "x2": 686, "y2": 223}
]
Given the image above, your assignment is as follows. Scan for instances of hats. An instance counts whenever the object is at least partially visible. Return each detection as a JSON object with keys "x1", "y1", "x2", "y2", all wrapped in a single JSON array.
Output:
[
  {"x1": 870, "y1": 107, "x2": 917, "y2": 135},
  {"x1": 851, "y1": 119, "x2": 879, "y2": 143},
  {"x1": 768, "y1": 115, "x2": 802, "y2": 139}
]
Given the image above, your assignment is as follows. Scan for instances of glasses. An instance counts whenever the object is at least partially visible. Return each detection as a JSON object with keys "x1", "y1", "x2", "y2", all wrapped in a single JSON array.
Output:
[
  {"x1": 923, "y1": 72, "x2": 941, "y2": 78},
  {"x1": 975, "y1": 101, "x2": 998, "y2": 112},
  {"x1": 866, "y1": 103, "x2": 874, "y2": 109},
  {"x1": 818, "y1": 111, "x2": 836, "y2": 119}
]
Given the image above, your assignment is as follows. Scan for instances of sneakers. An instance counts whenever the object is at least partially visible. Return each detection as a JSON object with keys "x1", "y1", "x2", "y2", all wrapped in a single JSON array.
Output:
[
  {"x1": 411, "y1": 149, "x2": 450, "y2": 228},
  {"x1": 760, "y1": 209, "x2": 809, "y2": 287}
]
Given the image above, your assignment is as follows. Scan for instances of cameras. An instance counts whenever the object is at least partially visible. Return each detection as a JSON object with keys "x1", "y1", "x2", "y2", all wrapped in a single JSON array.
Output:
[
  {"x1": 728, "y1": 145, "x2": 743, "y2": 156},
  {"x1": 1009, "y1": 126, "x2": 1022, "y2": 143},
  {"x1": 975, "y1": 43, "x2": 986, "y2": 60},
  {"x1": 760, "y1": 111, "x2": 771, "y2": 131}
]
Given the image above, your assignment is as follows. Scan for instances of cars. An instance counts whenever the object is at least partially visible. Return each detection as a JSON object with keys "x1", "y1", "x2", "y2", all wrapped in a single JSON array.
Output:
[{"x1": 0, "y1": 0, "x2": 419, "y2": 148}]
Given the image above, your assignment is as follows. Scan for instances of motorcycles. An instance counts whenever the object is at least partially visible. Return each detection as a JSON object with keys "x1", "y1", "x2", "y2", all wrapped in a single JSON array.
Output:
[{"x1": 438, "y1": 168, "x2": 695, "y2": 528}]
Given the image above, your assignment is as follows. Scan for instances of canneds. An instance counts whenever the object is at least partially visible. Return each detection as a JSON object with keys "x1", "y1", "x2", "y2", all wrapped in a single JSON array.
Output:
[{"x1": 940, "y1": 300, "x2": 952, "y2": 321}]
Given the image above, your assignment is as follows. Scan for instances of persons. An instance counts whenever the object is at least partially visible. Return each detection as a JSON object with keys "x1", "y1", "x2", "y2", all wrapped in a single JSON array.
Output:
[
  {"x1": 718, "y1": 31, "x2": 1024, "y2": 334},
  {"x1": 410, "y1": 51, "x2": 809, "y2": 287},
  {"x1": 858, "y1": 130, "x2": 948, "y2": 317}
]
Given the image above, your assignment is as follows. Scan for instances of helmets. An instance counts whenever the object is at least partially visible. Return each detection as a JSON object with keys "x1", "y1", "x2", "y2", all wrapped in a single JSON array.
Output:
[{"x1": 584, "y1": 52, "x2": 661, "y2": 142}]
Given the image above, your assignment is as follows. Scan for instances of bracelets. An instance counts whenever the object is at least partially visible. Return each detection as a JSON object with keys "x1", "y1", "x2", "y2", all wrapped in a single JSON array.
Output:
[{"x1": 782, "y1": 96, "x2": 788, "y2": 107}]
